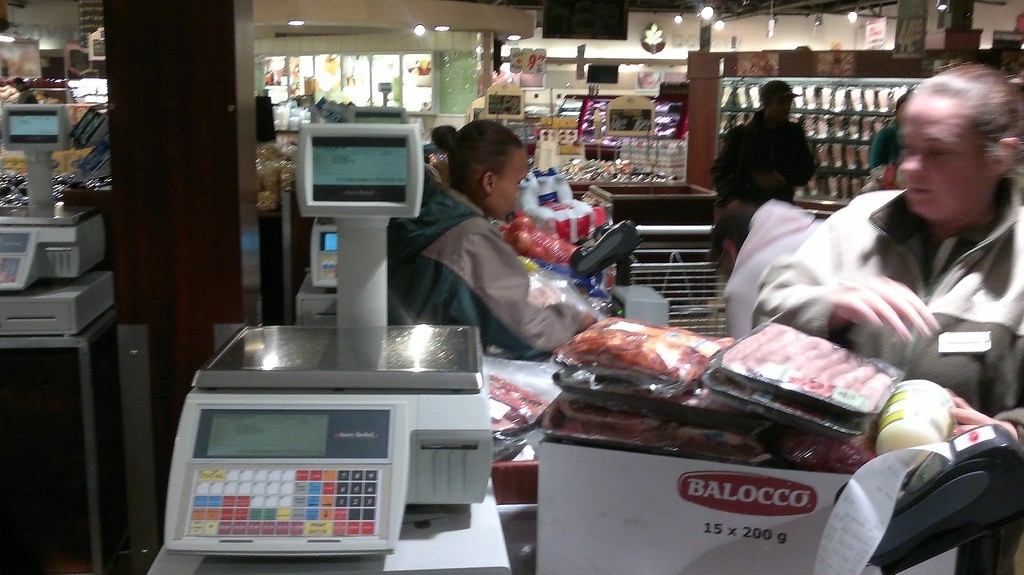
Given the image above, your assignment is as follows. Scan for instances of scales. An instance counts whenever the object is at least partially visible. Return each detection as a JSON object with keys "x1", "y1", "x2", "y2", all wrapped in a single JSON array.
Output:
[
  {"x1": 156, "y1": 120, "x2": 496, "y2": 560},
  {"x1": 1, "y1": 102, "x2": 110, "y2": 294}
]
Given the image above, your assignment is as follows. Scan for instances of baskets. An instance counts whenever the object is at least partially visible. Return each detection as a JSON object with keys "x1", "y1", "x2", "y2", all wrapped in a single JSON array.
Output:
[{"x1": 631, "y1": 249, "x2": 728, "y2": 337}]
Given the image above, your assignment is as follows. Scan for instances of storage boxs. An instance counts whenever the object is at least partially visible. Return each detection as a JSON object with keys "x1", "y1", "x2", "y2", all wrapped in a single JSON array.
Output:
[
  {"x1": 492, "y1": 420, "x2": 538, "y2": 461},
  {"x1": 543, "y1": 201, "x2": 592, "y2": 243},
  {"x1": 552, "y1": 369, "x2": 690, "y2": 412},
  {"x1": 713, "y1": 319, "x2": 905, "y2": 426},
  {"x1": 700, "y1": 367, "x2": 868, "y2": 441},
  {"x1": 534, "y1": 437, "x2": 959, "y2": 574},
  {"x1": 533, "y1": 400, "x2": 768, "y2": 467},
  {"x1": 553, "y1": 354, "x2": 710, "y2": 394}
]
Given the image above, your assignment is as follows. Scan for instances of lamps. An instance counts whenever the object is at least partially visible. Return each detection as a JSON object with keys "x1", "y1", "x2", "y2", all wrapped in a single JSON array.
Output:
[{"x1": 936, "y1": 0, "x2": 949, "y2": 14}]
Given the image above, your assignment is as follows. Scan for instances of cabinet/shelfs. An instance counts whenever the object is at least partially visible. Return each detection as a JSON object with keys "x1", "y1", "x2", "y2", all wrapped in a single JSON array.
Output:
[
  {"x1": 577, "y1": 95, "x2": 687, "y2": 162},
  {"x1": 714, "y1": 76, "x2": 920, "y2": 216}
]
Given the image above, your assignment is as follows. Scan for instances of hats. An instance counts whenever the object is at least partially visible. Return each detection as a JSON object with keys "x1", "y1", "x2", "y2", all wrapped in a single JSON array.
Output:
[{"x1": 760, "y1": 80, "x2": 799, "y2": 102}]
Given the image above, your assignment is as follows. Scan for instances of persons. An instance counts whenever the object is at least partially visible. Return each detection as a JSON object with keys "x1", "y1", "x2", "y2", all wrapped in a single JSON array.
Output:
[
  {"x1": 387, "y1": 118, "x2": 597, "y2": 360},
  {"x1": 752, "y1": 62, "x2": 1024, "y2": 445},
  {"x1": 711, "y1": 199, "x2": 824, "y2": 340},
  {"x1": 869, "y1": 93, "x2": 908, "y2": 168},
  {"x1": 13, "y1": 77, "x2": 38, "y2": 104},
  {"x1": 712, "y1": 81, "x2": 817, "y2": 215}
]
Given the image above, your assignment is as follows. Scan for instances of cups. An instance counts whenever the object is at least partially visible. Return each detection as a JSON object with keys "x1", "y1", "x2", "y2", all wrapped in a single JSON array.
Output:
[
  {"x1": 874, "y1": 379, "x2": 958, "y2": 458},
  {"x1": 272, "y1": 106, "x2": 312, "y2": 130}
]
